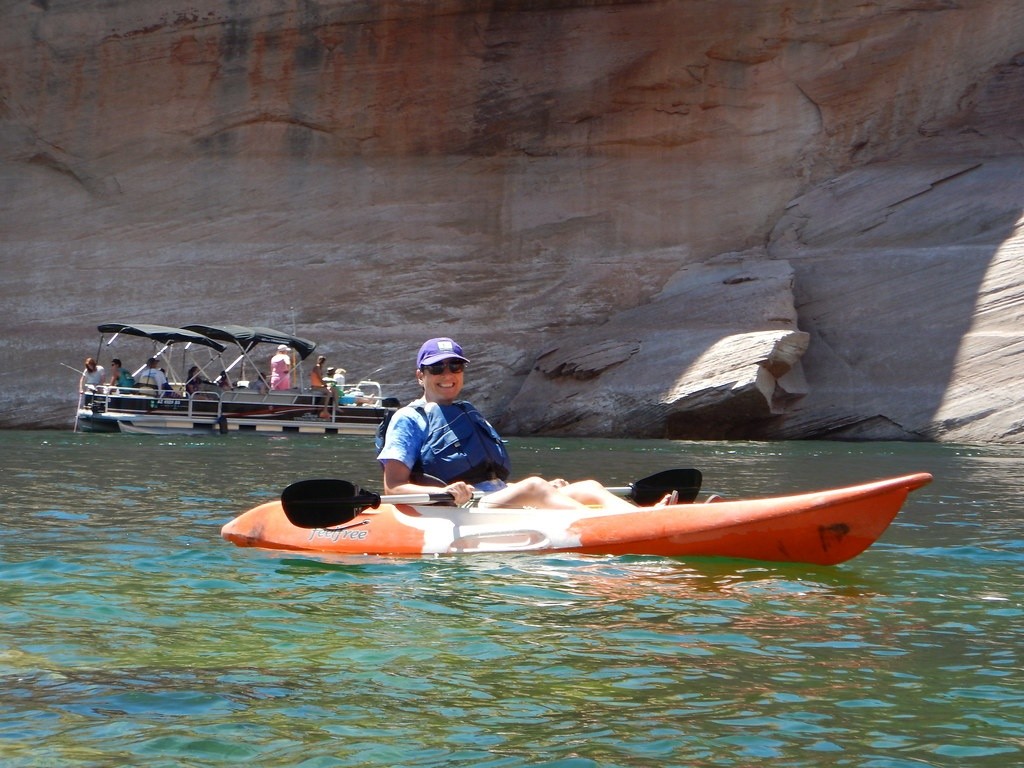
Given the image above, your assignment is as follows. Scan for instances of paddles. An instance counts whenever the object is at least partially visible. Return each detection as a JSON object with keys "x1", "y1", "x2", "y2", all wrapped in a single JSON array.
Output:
[{"x1": 278, "y1": 466, "x2": 704, "y2": 530}]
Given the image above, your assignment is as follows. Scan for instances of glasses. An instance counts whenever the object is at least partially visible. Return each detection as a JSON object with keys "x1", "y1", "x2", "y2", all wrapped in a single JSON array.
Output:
[
  {"x1": 111, "y1": 363, "x2": 115, "y2": 367},
  {"x1": 424, "y1": 358, "x2": 466, "y2": 375}
]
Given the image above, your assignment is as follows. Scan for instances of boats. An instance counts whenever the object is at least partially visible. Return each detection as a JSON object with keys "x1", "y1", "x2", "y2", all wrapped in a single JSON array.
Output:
[
  {"x1": 72, "y1": 322, "x2": 402, "y2": 435},
  {"x1": 220, "y1": 465, "x2": 933, "y2": 567}
]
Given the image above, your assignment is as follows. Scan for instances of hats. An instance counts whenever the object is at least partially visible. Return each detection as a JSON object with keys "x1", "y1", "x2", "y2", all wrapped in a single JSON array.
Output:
[
  {"x1": 277, "y1": 343, "x2": 292, "y2": 352},
  {"x1": 318, "y1": 355, "x2": 326, "y2": 361},
  {"x1": 326, "y1": 366, "x2": 336, "y2": 372},
  {"x1": 416, "y1": 336, "x2": 471, "y2": 369},
  {"x1": 146, "y1": 357, "x2": 160, "y2": 366}
]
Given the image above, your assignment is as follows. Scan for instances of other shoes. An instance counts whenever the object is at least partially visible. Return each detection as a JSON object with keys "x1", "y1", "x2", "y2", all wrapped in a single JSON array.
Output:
[
  {"x1": 319, "y1": 410, "x2": 332, "y2": 418},
  {"x1": 336, "y1": 408, "x2": 344, "y2": 413}
]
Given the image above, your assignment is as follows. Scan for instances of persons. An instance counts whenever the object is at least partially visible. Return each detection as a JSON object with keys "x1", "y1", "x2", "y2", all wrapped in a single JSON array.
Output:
[
  {"x1": 219, "y1": 371, "x2": 246, "y2": 389},
  {"x1": 311, "y1": 356, "x2": 326, "y2": 385},
  {"x1": 250, "y1": 373, "x2": 270, "y2": 389},
  {"x1": 186, "y1": 367, "x2": 216, "y2": 399},
  {"x1": 270, "y1": 344, "x2": 291, "y2": 390},
  {"x1": 79, "y1": 358, "x2": 105, "y2": 394},
  {"x1": 324, "y1": 367, "x2": 374, "y2": 403},
  {"x1": 109, "y1": 359, "x2": 135, "y2": 393},
  {"x1": 376, "y1": 337, "x2": 676, "y2": 509},
  {"x1": 141, "y1": 359, "x2": 166, "y2": 396},
  {"x1": 158, "y1": 368, "x2": 180, "y2": 396}
]
